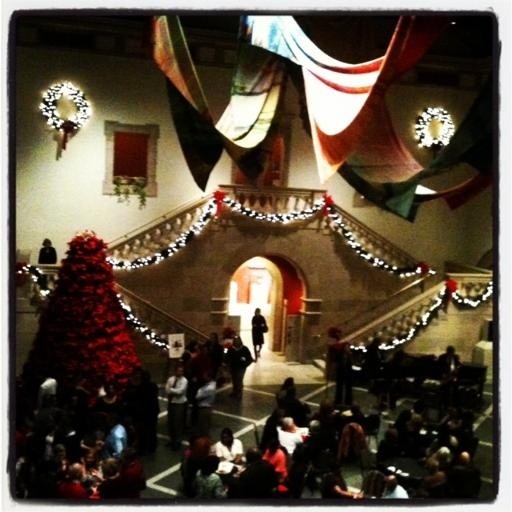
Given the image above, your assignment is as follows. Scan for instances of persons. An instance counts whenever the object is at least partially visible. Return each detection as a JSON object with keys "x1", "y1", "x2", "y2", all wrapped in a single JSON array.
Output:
[
  {"x1": 15, "y1": 369, "x2": 161, "y2": 499},
  {"x1": 252, "y1": 309, "x2": 266, "y2": 358},
  {"x1": 38, "y1": 239, "x2": 57, "y2": 265},
  {"x1": 165, "y1": 332, "x2": 253, "y2": 452},
  {"x1": 443, "y1": 275, "x2": 458, "y2": 293},
  {"x1": 327, "y1": 329, "x2": 465, "y2": 422},
  {"x1": 181, "y1": 377, "x2": 473, "y2": 500}
]
